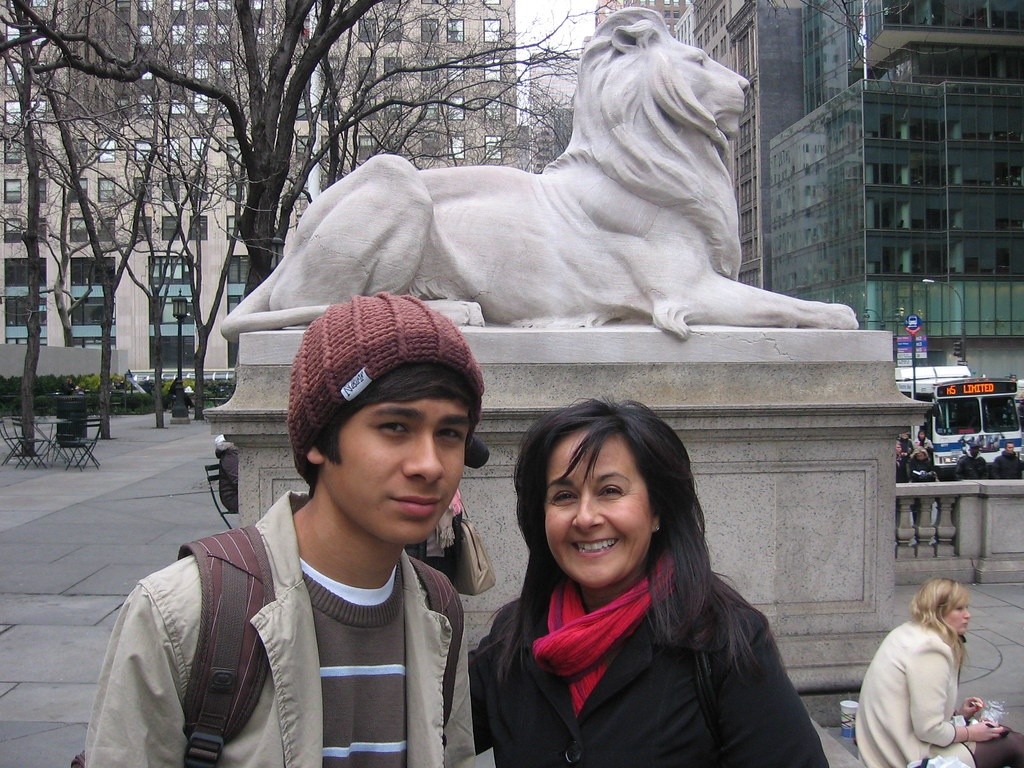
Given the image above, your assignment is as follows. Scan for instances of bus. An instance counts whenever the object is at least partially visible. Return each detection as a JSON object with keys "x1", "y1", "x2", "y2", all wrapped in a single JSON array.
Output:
[{"x1": 896, "y1": 375, "x2": 1024, "y2": 480}]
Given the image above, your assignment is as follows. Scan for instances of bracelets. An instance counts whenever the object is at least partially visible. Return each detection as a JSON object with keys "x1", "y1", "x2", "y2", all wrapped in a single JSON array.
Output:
[{"x1": 965, "y1": 726, "x2": 969, "y2": 742}]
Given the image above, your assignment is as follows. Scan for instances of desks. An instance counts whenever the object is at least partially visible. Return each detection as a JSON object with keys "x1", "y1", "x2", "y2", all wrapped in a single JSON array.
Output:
[
  {"x1": 33, "y1": 419, "x2": 72, "y2": 461},
  {"x1": 204, "y1": 397, "x2": 229, "y2": 406}
]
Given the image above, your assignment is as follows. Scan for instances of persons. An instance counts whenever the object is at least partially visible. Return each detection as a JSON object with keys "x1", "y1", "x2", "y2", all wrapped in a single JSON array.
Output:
[
  {"x1": 214, "y1": 434, "x2": 238, "y2": 512},
  {"x1": 895, "y1": 375, "x2": 1024, "y2": 483},
  {"x1": 403, "y1": 430, "x2": 489, "y2": 592},
  {"x1": 855, "y1": 578, "x2": 1024, "y2": 768},
  {"x1": 170, "y1": 378, "x2": 195, "y2": 413},
  {"x1": 84, "y1": 290, "x2": 486, "y2": 768},
  {"x1": 468, "y1": 393, "x2": 829, "y2": 768}
]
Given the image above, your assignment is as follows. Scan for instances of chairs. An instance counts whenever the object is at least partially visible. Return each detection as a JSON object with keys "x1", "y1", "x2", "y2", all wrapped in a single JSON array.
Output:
[
  {"x1": 0, "y1": 417, "x2": 104, "y2": 471},
  {"x1": 205, "y1": 464, "x2": 239, "y2": 529}
]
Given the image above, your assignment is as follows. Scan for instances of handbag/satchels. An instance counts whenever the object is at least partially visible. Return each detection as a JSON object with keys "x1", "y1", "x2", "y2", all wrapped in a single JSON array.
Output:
[
  {"x1": 919, "y1": 471, "x2": 936, "y2": 482},
  {"x1": 449, "y1": 520, "x2": 496, "y2": 596}
]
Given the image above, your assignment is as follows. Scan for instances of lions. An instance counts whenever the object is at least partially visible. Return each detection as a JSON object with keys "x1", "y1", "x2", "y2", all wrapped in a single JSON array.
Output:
[{"x1": 218, "y1": 7, "x2": 858, "y2": 343}]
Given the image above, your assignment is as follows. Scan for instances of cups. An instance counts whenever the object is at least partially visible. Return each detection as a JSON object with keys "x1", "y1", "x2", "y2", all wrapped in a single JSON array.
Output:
[{"x1": 840, "y1": 699, "x2": 858, "y2": 739}]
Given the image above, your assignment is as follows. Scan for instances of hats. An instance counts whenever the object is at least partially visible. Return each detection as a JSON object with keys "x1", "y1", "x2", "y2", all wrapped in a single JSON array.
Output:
[
  {"x1": 970, "y1": 443, "x2": 981, "y2": 449},
  {"x1": 287, "y1": 291, "x2": 485, "y2": 482}
]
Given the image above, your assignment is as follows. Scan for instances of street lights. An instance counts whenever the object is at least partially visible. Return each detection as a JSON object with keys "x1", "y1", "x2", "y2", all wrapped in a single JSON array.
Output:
[
  {"x1": 862, "y1": 307, "x2": 905, "y2": 331},
  {"x1": 922, "y1": 279, "x2": 967, "y2": 366},
  {"x1": 171, "y1": 288, "x2": 191, "y2": 425}
]
